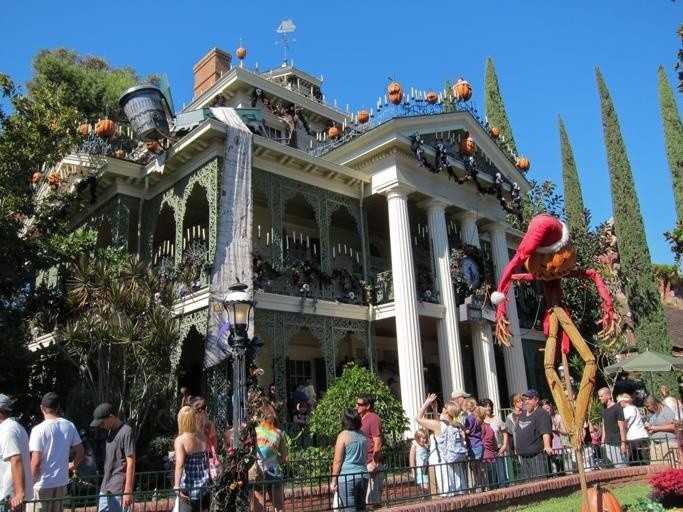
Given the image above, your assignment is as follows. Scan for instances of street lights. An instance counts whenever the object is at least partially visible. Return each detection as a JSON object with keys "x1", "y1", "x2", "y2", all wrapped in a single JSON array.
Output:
[{"x1": 221, "y1": 274, "x2": 257, "y2": 452}]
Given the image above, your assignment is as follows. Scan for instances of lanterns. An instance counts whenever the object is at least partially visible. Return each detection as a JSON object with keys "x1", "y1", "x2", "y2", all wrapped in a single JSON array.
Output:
[
  {"x1": 236, "y1": 47, "x2": 246, "y2": 59},
  {"x1": 30, "y1": 114, "x2": 160, "y2": 186},
  {"x1": 324, "y1": 73, "x2": 531, "y2": 172}
]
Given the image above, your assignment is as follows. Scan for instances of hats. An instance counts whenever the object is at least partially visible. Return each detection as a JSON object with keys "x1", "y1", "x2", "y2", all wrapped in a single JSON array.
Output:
[
  {"x1": 616, "y1": 394, "x2": 630, "y2": 406},
  {"x1": 0, "y1": 393, "x2": 20, "y2": 416},
  {"x1": 41, "y1": 391, "x2": 60, "y2": 410},
  {"x1": 521, "y1": 389, "x2": 540, "y2": 396},
  {"x1": 89, "y1": 403, "x2": 115, "y2": 427},
  {"x1": 451, "y1": 389, "x2": 472, "y2": 398}
]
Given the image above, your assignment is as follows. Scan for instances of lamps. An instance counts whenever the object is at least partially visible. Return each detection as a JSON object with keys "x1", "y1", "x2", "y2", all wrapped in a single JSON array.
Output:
[{"x1": 114, "y1": 83, "x2": 186, "y2": 155}]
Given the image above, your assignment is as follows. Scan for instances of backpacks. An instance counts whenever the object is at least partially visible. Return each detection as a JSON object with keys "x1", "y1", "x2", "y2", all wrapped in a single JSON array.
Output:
[{"x1": 434, "y1": 420, "x2": 469, "y2": 464}]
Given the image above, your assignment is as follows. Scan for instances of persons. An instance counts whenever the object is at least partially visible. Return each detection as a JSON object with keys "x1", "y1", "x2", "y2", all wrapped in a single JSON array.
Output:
[
  {"x1": 491, "y1": 214, "x2": 618, "y2": 448},
  {"x1": 331, "y1": 393, "x2": 384, "y2": 511},
  {"x1": 409, "y1": 387, "x2": 683, "y2": 503},
  {"x1": 212, "y1": 375, "x2": 327, "y2": 439},
  {"x1": 0, "y1": 392, "x2": 84, "y2": 511},
  {"x1": 90, "y1": 403, "x2": 136, "y2": 512},
  {"x1": 173, "y1": 396, "x2": 289, "y2": 511},
  {"x1": 456, "y1": 251, "x2": 480, "y2": 296},
  {"x1": 293, "y1": 401, "x2": 310, "y2": 450}
]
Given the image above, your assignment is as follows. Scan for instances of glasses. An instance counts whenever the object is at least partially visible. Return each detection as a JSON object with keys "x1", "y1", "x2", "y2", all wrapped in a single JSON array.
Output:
[{"x1": 357, "y1": 403, "x2": 365, "y2": 406}]
